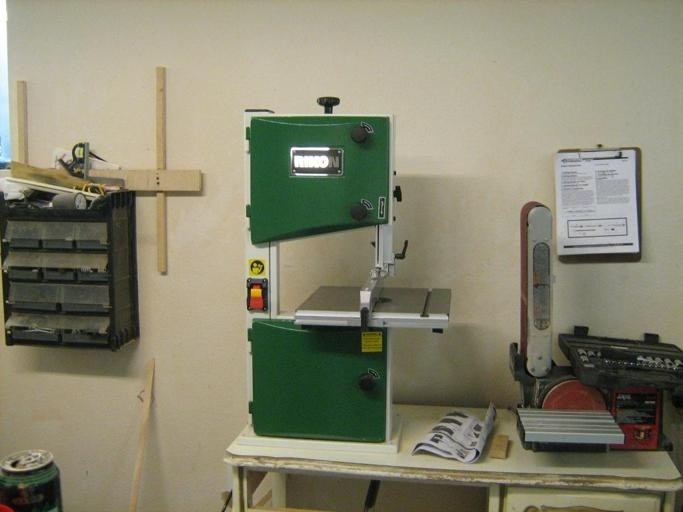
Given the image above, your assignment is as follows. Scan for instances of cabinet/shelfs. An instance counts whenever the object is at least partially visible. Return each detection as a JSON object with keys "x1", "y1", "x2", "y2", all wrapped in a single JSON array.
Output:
[{"x1": 220, "y1": 403, "x2": 683, "y2": 512}]
